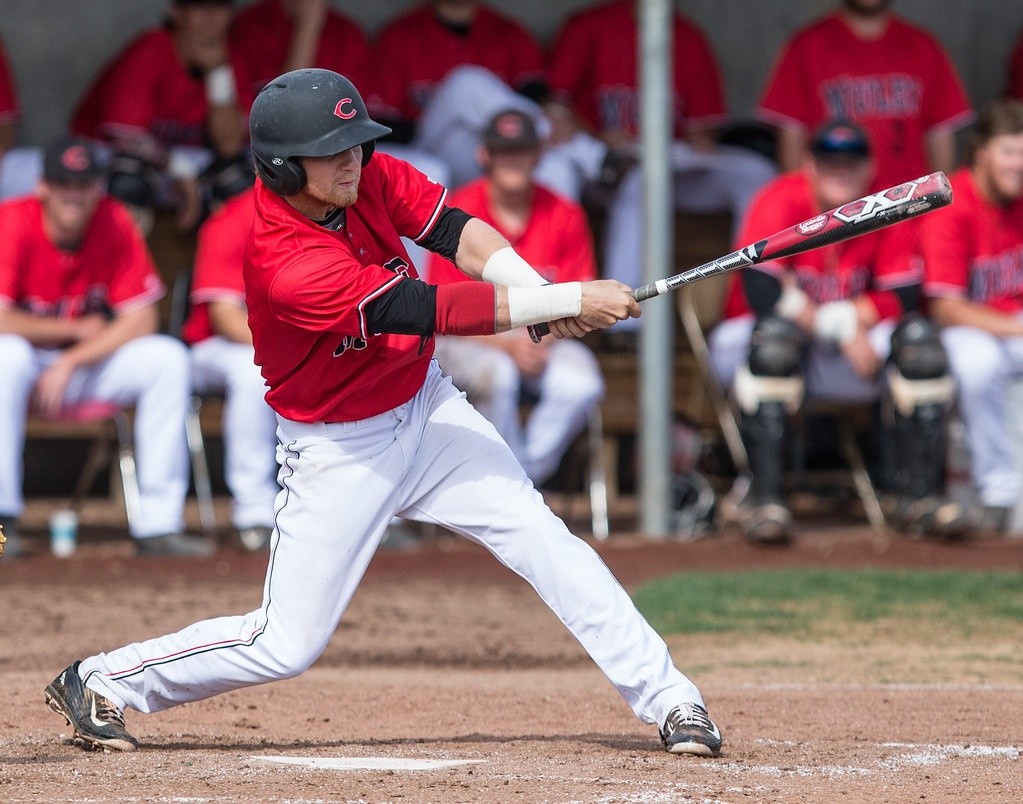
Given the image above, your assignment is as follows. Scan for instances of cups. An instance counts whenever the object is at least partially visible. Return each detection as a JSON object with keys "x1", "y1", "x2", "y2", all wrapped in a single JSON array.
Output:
[{"x1": 48, "y1": 509, "x2": 79, "y2": 556}]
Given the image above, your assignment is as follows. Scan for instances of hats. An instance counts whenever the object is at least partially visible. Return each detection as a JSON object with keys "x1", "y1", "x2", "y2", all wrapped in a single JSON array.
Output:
[
  {"x1": 43, "y1": 131, "x2": 114, "y2": 183},
  {"x1": 487, "y1": 109, "x2": 538, "y2": 149},
  {"x1": 814, "y1": 118, "x2": 872, "y2": 162}
]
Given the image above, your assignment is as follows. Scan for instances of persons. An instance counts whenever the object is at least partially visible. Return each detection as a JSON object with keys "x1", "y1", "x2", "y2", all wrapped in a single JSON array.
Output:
[
  {"x1": 921, "y1": 105, "x2": 1023, "y2": 538},
  {"x1": 712, "y1": 122, "x2": 969, "y2": 542},
  {"x1": 374, "y1": 0, "x2": 626, "y2": 200},
  {"x1": 0, "y1": 139, "x2": 205, "y2": 555},
  {"x1": 760, "y1": 0, "x2": 976, "y2": 182},
  {"x1": 44, "y1": 68, "x2": 722, "y2": 755},
  {"x1": 175, "y1": 157, "x2": 281, "y2": 549},
  {"x1": 428, "y1": 111, "x2": 604, "y2": 484},
  {"x1": 0, "y1": 0, "x2": 378, "y2": 238},
  {"x1": 555, "y1": 0, "x2": 779, "y2": 330}
]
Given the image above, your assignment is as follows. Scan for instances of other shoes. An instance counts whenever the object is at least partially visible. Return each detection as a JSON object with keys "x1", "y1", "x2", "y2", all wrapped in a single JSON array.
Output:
[
  {"x1": 136, "y1": 529, "x2": 219, "y2": 555},
  {"x1": 219, "y1": 526, "x2": 271, "y2": 553},
  {"x1": 747, "y1": 504, "x2": 794, "y2": 544},
  {"x1": 903, "y1": 493, "x2": 970, "y2": 538},
  {"x1": 0, "y1": 520, "x2": 32, "y2": 557},
  {"x1": 378, "y1": 527, "x2": 415, "y2": 547}
]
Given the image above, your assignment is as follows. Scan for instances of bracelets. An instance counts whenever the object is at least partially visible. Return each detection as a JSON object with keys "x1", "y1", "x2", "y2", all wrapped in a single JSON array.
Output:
[
  {"x1": 482, "y1": 247, "x2": 550, "y2": 286},
  {"x1": 169, "y1": 154, "x2": 196, "y2": 180},
  {"x1": 206, "y1": 64, "x2": 236, "y2": 106},
  {"x1": 507, "y1": 282, "x2": 582, "y2": 329}
]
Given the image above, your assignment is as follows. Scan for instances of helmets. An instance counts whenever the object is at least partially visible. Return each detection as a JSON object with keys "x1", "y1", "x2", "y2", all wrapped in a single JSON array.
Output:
[{"x1": 249, "y1": 69, "x2": 394, "y2": 196}]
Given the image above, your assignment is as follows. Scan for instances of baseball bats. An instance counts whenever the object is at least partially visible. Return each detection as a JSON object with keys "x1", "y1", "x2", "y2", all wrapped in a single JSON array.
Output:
[{"x1": 519, "y1": 172, "x2": 953, "y2": 345}]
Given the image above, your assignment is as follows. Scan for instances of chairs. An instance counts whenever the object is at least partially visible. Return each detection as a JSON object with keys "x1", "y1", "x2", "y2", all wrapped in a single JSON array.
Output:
[
  {"x1": 168, "y1": 258, "x2": 233, "y2": 541},
  {"x1": 16, "y1": 417, "x2": 128, "y2": 530},
  {"x1": 679, "y1": 275, "x2": 890, "y2": 532}
]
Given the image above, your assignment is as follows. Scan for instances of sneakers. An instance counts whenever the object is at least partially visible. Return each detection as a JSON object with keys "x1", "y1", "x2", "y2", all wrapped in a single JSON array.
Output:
[
  {"x1": 44, "y1": 656, "x2": 139, "y2": 753},
  {"x1": 657, "y1": 702, "x2": 722, "y2": 758}
]
